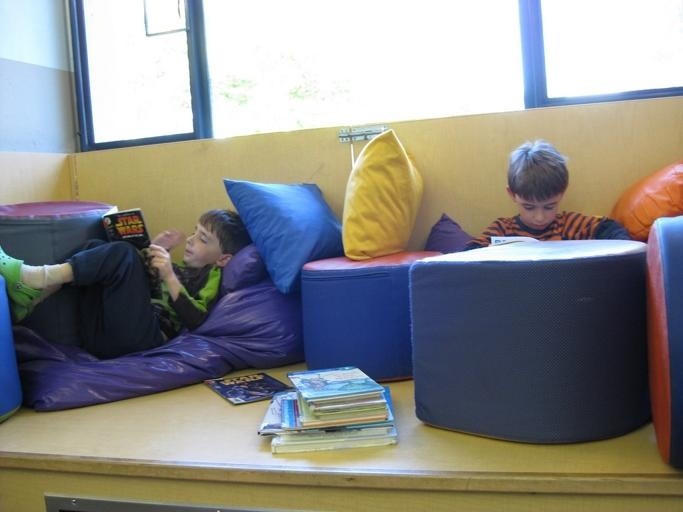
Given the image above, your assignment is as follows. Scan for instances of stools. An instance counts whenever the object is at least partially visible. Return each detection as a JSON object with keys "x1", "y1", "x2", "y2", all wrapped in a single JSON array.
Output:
[
  {"x1": 301, "y1": 250, "x2": 447, "y2": 384},
  {"x1": 0, "y1": 198, "x2": 119, "y2": 264},
  {"x1": 0, "y1": 274, "x2": 25, "y2": 423},
  {"x1": 642, "y1": 214, "x2": 682, "y2": 468},
  {"x1": 408, "y1": 238, "x2": 649, "y2": 445}
]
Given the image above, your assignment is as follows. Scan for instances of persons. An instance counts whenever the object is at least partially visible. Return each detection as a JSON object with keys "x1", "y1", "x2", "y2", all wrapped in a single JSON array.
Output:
[
  {"x1": 458, "y1": 141, "x2": 631, "y2": 254},
  {"x1": 0, "y1": 208, "x2": 253, "y2": 360}
]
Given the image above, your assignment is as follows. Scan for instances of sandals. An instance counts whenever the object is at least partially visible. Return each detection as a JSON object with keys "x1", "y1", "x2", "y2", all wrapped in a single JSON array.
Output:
[
  {"x1": 0, "y1": 246, "x2": 42, "y2": 307},
  {"x1": 10, "y1": 302, "x2": 28, "y2": 323}
]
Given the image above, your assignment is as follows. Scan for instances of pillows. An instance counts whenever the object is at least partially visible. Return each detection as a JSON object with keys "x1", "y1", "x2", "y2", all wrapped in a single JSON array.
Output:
[
  {"x1": 424, "y1": 210, "x2": 482, "y2": 256},
  {"x1": 222, "y1": 177, "x2": 344, "y2": 296},
  {"x1": 610, "y1": 159, "x2": 682, "y2": 241},
  {"x1": 341, "y1": 128, "x2": 423, "y2": 261}
]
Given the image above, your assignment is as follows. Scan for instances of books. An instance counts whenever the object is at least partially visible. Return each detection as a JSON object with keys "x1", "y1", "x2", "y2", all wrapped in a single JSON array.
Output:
[
  {"x1": 489, "y1": 235, "x2": 544, "y2": 247},
  {"x1": 103, "y1": 205, "x2": 162, "y2": 299},
  {"x1": 203, "y1": 372, "x2": 288, "y2": 405},
  {"x1": 257, "y1": 361, "x2": 401, "y2": 457}
]
